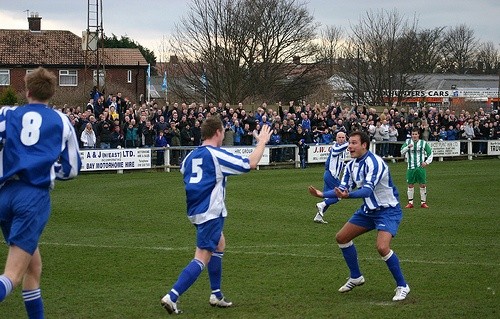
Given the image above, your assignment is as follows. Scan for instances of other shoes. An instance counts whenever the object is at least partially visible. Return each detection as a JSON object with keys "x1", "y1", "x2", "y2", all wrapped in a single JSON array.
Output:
[
  {"x1": 314, "y1": 213, "x2": 328, "y2": 224},
  {"x1": 315, "y1": 202, "x2": 326, "y2": 217},
  {"x1": 405, "y1": 203, "x2": 414, "y2": 208},
  {"x1": 161, "y1": 294, "x2": 184, "y2": 315},
  {"x1": 210, "y1": 294, "x2": 232, "y2": 308},
  {"x1": 421, "y1": 203, "x2": 429, "y2": 208}
]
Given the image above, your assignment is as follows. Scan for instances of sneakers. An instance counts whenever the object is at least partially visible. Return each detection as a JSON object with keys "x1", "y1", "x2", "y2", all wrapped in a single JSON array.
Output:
[
  {"x1": 338, "y1": 276, "x2": 365, "y2": 293},
  {"x1": 392, "y1": 284, "x2": 410, "y2": 301}
]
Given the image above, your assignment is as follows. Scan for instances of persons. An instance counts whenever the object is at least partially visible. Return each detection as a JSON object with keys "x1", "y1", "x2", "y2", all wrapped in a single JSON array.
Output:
[
  {"x1": 52, "y1": 86, "x2": 500, "y2": 169},
  {"x1": 306, "y1": 131, "x2": 410, "y2": 301},
  {"x1": 0, "y1": 67, "x2": 82, "y2": 319},
  {"x1": 313, "y1": 132, "x2": 350, "y2": 224},
  {"x1": 160, "y1": 115, "x2": 274, "y2": 314},
  {"x1": 400, "y1": 129, "x2": 434, "y2": 208}
]
